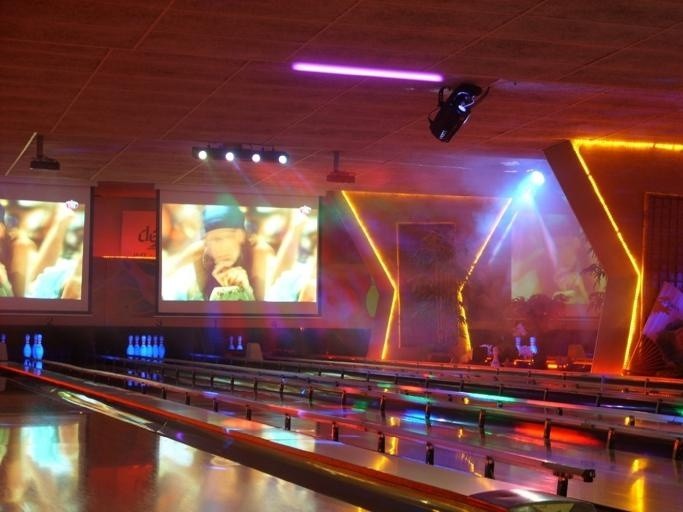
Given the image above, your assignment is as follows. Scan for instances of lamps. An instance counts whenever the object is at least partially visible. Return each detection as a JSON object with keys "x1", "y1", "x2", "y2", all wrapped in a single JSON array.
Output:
[
  {"x1": 192, "y1": 145, "x2": 289, "y2": 165},
  {"x1": 427, "y1": 81, "x2": 484, "y2": 143}
]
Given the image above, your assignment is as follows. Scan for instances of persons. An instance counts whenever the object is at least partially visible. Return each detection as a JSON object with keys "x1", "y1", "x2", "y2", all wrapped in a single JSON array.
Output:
[
  {"x1": 184, "y1": 206, "x2": 309, "y2": 300},
  {"x1": 0, "y1": 202, "x2": 70, "y2": 298}
]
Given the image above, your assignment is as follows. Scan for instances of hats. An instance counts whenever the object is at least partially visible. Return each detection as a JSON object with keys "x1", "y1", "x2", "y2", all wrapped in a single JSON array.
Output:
[{"x1": 203, "y1": 207, "x2": 245, "y2": 230}]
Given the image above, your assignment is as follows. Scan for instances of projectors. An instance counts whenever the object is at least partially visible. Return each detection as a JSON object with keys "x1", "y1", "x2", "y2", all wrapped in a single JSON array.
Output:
[
  {"x1": 30, "y1": 161, "x2": 60, "y2": 171},
  {"x1": 326, "y1": 174, "x2": 355, "y2": 183}
]
